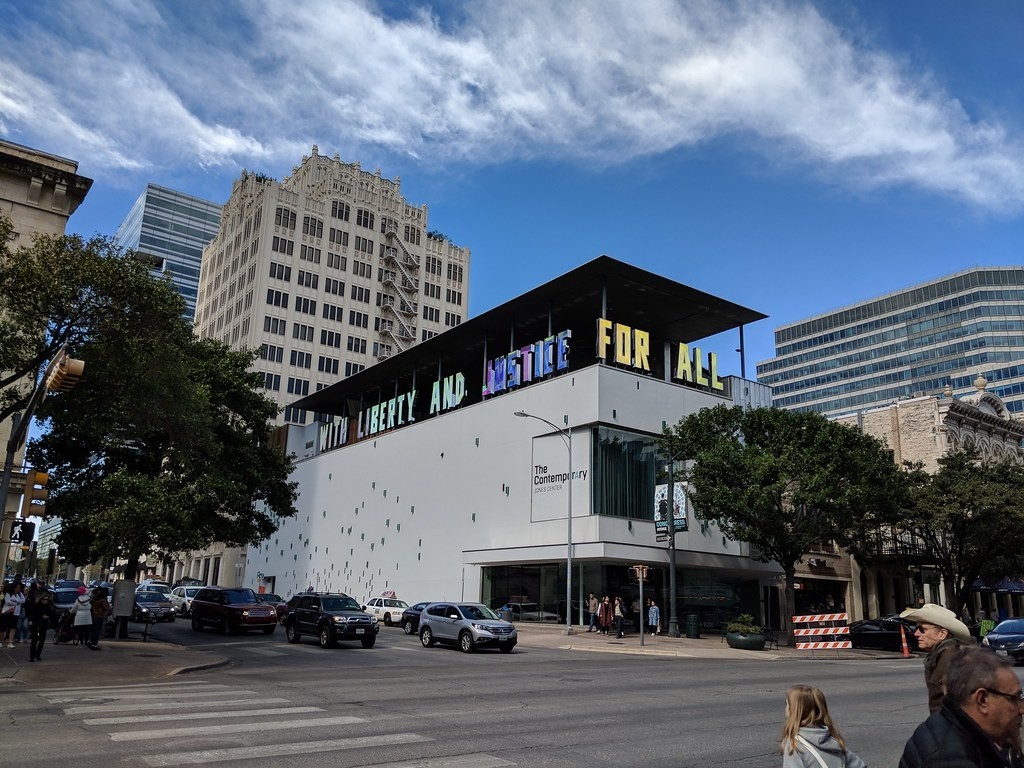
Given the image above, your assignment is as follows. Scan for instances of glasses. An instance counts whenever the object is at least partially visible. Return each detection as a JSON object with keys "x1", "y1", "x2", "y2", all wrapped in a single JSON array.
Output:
[
  {"x1": 914, "y1": 625, "x2": 940, "y2": 634},
  {"x1": 42, "y1": 599, "x2": 49, "y2": 602},
  {"x1": 971, "y1": 687, "x2": 1024, "y2": 705}
]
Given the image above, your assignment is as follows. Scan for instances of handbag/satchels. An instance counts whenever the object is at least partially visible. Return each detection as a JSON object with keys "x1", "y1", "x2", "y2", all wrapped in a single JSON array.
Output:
[{"x1": 657, "y1": 620, "x2": 662, "y2": 632}]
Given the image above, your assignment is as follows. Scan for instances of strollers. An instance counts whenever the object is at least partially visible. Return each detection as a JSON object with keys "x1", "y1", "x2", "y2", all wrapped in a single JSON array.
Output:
[{"x1": 53, "y1": 614, "x2": 79, "y2": 646}]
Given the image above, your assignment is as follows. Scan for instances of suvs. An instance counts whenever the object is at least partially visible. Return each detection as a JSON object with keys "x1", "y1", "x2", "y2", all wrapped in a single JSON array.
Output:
[
  {"x1": 54, "y1": 579, "x2": 86, "y2": 588},
  {"x1": 285, "y1": 592, "x2": 379, "y2": 648},
  {"x1": 419, "y1": 601, "x2": 518, "y2": 654}
]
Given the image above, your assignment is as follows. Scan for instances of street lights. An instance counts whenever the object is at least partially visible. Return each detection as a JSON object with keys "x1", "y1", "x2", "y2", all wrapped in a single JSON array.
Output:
[
  {"x1": 668, "y1": 428, "x2": 729, "y2": 637},
  {"x1": 514, "y1": 412, "x2": 572, "y2": 627}
]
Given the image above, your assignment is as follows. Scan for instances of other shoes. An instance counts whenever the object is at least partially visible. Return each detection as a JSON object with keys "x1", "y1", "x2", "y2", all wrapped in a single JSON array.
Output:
[
  {"x1": 606, "y1": 634, "x2": 609, "y2": 636},
  {"x1": 78, "y1": 642, "x2": 101, "y2": 651},
  {"x1": 7, "y1": 644, "x2": 15, "y2": 649},
  {"x1": 34, "y1": 655, "x2": 41, "y2": 660},
  {"x1": 0, "y1": 643, "x2": 3, "y2": 647},
  {"x1": 586, "y1": 630, "x2": 592, "y2": 632},
  {"x1": 29, "y1": 657, "x2": 33, "y2": 662},
  {"x1": 622, "y1": 632, "x2": 625, "y2": 635},
  {"x1": 650, "y1": 633, "x2": 656, "y2": 636},
  {"x1": 602, "y1": 633, "x2": 604, "y2": 636},
  {"x1": 617, "y1": 635, "x2": 619, "y2": 639}
]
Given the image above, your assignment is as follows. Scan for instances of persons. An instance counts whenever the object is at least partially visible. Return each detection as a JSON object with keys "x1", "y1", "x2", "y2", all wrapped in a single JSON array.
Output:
[
  {"x1": 585, "y1": 592, "x2": 660, "y2": 638},
  {"x1": 900, "y1": 603, "x2": 1024, "y2": 768},
  {"x1": 779, "y1": 684, "x2": 867, "y2": 768},
  {"x1": 0, "y1": 574, "x2": 112, "y2": 663}
]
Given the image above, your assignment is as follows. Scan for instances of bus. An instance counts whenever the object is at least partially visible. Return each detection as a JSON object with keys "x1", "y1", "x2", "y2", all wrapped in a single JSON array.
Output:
[{"x1": 189, "y1": 586, "x2": 277, "y2": 635}]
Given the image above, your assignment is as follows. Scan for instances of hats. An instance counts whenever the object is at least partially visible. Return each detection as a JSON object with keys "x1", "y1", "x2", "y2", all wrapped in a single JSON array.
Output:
[
  {"x1": 37, "y1": 580, "x2": 45, "y2": 588},
  {"x1": 78, "y1": 586, "x2": 85, "y2": 595},
  {"x1": 900, "y1": 603, "x2": 970, "y2": 640}
]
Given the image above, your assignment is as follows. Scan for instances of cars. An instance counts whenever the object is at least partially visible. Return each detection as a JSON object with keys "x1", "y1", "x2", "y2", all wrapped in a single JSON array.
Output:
[
  {"x1": 981, "y1": 619, "x2": 1024, "y2": 667},
  {"x1": 48, "y1": 588, "x2": 78, "y2": 611},
  {"x1": 825, "y1": 613, "x2": 920, "y2": 652},
  {"x1": 168, "y1": 586, "x2": 203, "y2": 616},
  {"x1": 132, "y1": 591, "x2": 177, "y2": 623},
  {"x1": 88, "y1": 581, "x2": 114, "y2": 603},
  {"x1": 256, "y1": 593, "x2": 287, "y2": 627},
  {"x1": 135, "y1": 584, "x2": 173, "y2": 602},
  {"x1": 359, "y1": 597, "x2": 434, "y2": 635},
  {"x1": 6, "y1": 575, "x2": 40, "y2": 587}
]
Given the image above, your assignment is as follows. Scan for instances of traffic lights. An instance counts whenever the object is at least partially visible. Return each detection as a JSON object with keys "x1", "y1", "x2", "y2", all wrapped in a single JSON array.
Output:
[
  {"x1": 9, "y1": 469, "x2": 48, "y2": 541},
  {"x1": 628, "y1": 567, "x2": 639, "y2": 580},
  {"x1": 46, "y1": 355, "x2": 85, "y2": 394}
]
